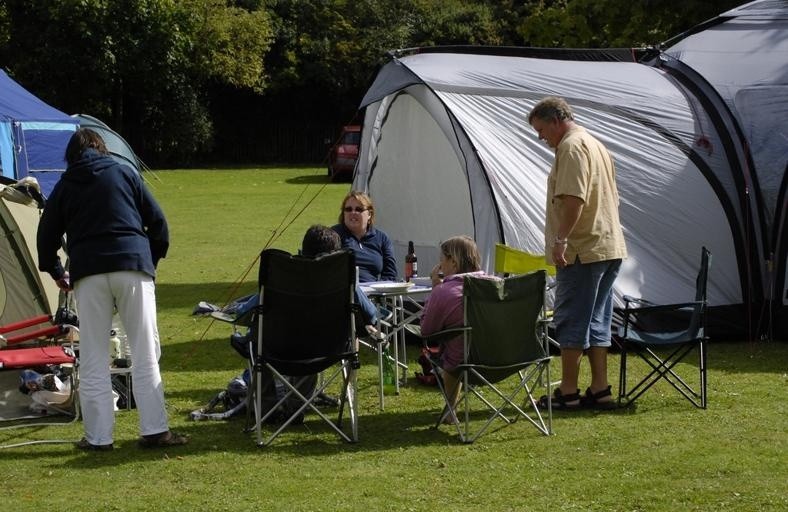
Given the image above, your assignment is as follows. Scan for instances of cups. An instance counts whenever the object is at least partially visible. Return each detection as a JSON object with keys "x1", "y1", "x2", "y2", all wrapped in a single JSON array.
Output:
[
  {"x1": 110, "y1": 329, "x2": 121, "y2": 369},
  {"x1": 430, "y1": 268, "x2": 445, "y2": 280},
  {"x1": 124, "y1": 335, "x2": 133, "y2": 367}
]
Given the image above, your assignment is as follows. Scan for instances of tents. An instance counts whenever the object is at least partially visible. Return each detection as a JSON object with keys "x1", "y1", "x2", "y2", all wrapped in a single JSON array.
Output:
[
  {"x1": 1, "y1": 174, "x2": 80, "y2": 346},
  {"x1": 0, "y1": 67, "x2": 80, "y2": 203},
  {"x1": 351, "y1": 0, "x2": 788, "y2": 346},
  {"x1": 72, "y1": 112, "x2": 142, "y2": 176}
]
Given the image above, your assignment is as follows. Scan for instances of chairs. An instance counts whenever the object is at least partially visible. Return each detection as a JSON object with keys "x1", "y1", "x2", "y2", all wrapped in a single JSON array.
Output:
[
  {"x1": 494, "y1": 244, "x2": 561, "y2": 387},
  {"x1": 422, "y1": 269, "x2": 553, "y2": 442},
  {"x1": 383, "y1": 296, "x2": 408, "y2": 380},
  {"x1": 248, "y1": 247, "x2": 362, "y2": 447},
  {"x1": 619, "y1": 245, "x2": 711, "y2": 409},
  {"x1": 0, "y1": 279, "x2": 87, "y2": 450}
]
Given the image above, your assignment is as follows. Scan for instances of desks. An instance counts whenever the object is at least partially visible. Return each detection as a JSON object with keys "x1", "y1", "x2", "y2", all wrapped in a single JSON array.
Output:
[{"x1": 358, "y1": 277, "x2": 441, "y2": 410}]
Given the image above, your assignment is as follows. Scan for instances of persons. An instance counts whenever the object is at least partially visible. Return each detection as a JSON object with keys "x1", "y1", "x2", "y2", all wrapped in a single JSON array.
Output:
[
  {"x1": 527, "y1": 96, "x2": 627, "y2": 412},
  {"x1": 229, "y1": 224, "x2": 378, "y2": 422},
  {"x1": 37, "y1": 129, "x2": 188, "y2": 452},
  {"x1": 421, "y1": 236, "x2": 505, "y2": 424},
  {"x1": 332, "y1": 190, "x2": 397, "y2": 337}
]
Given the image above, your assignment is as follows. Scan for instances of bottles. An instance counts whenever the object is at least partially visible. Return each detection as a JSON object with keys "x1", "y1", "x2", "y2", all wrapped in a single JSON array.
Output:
[
  {"x1": 383, "y1": 348, "x2": 396, "y2": 397},
  {"x1": 405, "y1": 241, "x2": 418, "y2": 283}
]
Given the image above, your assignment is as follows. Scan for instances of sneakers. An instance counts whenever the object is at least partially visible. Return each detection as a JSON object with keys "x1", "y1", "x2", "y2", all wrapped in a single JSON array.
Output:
[
  {"x1": 231, "y1": 332, "x2": 250, "y2": 359},
  {"x1": 446, "y1": 410, "x2": 460, "y2": 425}
]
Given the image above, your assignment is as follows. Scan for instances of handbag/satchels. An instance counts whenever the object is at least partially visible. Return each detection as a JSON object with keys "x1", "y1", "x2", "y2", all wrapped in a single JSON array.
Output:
[{"x1": 223, "y1": 368, "x2": 317, "y2": 423}]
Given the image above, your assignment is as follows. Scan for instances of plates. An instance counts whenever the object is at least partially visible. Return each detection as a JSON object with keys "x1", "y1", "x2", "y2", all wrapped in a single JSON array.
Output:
[{"x1": 369, "y1": 283, "x2": 415, "y2": 293}]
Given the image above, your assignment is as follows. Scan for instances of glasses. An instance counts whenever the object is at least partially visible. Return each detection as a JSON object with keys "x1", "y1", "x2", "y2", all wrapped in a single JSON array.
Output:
[{"x1": 342, "y1": 207, "x2": 367, "y2": 212}]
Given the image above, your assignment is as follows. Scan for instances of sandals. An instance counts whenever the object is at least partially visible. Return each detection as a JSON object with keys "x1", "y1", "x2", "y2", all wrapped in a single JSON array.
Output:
[
  {"x1": 138, "y1": 430, "x2": 188, "y2": 450},
  {"x1": 530, "y1": 388, "x2": 581, "y2": 411},
  {"x1": 582, "y1": 385, "x2": 615, "y2": 411},
  {"x1": 74, "y1": 437, "x2": 113, "y2": 451}
]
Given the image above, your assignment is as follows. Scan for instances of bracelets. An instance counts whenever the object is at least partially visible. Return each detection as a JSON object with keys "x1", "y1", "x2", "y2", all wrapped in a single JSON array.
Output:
[{"x1": 553, "y1": 236, "x2": 567, "y2": 246}]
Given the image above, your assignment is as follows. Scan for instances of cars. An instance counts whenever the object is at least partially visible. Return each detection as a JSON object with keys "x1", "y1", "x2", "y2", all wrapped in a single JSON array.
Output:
[{"x1": 326, "y1": 124, "x2": 362, "y2": 182}]
